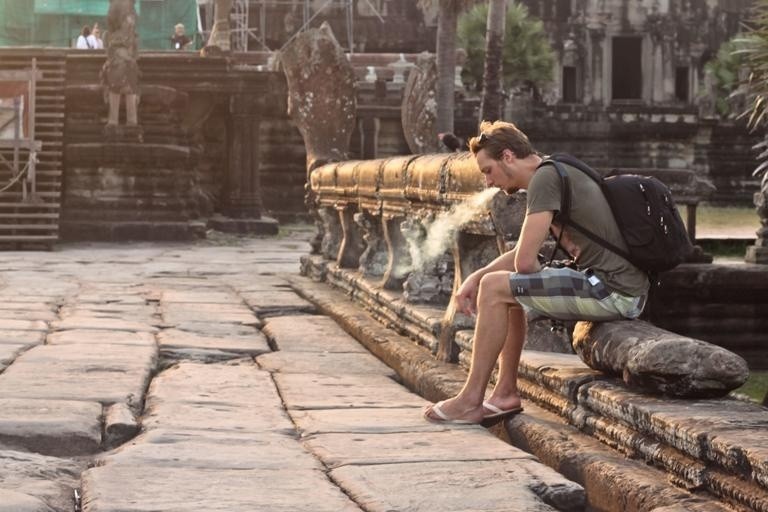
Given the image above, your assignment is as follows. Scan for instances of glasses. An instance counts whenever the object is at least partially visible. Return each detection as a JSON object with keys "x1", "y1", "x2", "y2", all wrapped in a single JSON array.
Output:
[{"x1": 478, "y1": 132, "x2": 515, "y2": 152}]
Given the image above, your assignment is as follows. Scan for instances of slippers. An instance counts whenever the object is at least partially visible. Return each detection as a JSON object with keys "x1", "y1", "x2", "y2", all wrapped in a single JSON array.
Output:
[
  {"x1": 480, "y1": 398, "x2": 525, "y2": 427},
  {"x1": 422, "y1": 397, "x2": 484, "y2": 425}
]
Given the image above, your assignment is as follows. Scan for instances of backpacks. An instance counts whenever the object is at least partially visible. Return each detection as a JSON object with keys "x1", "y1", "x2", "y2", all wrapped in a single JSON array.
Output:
[{"x1": 536, "y1": 149, "x2": 697, "y2": 277}]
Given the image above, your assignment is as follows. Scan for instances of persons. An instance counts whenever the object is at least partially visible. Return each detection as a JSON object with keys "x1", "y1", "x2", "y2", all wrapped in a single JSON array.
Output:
[
  {"x1": 422, "y1": 122, "x2": 651, "y2": 425},
  {"x1": 170, "y1": 23, "x2": 192, "y2": 50},
  {"x1": 74, "y1": 21, "x2": 104, "y2": 49}
]
